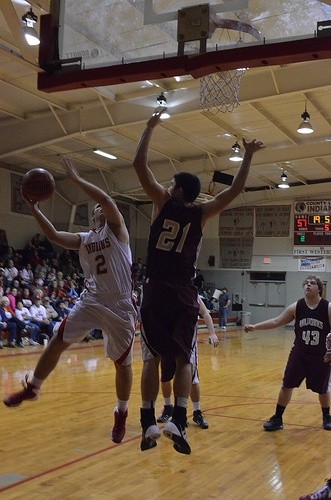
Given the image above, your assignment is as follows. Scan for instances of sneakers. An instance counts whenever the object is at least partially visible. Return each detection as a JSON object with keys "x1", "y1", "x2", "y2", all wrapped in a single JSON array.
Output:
[
  {"x1": 262, "y1": 414, "x2": 284, "y2": 430},
  {"x1": 140, "y1": 420, "x2": 162, "y2": 451},
  {"x1": 192, "y1": 410, "x2": 209, "y2": 429},
  {"x1": 298, "y1": 477, "x2": 331, "y2": 500},
  {"x1": 322, "y1": 414, "x2": 331, "y2": 430},
  {"x1": 157, "y1": 404, "x2": 174, "y2": 423},
  {"x1": 3, "y1": 374, "x2": 41, "y2": 407},
  {"x1": 162, "y1": 419, "x2": 192, "y2": 456},
  {"x1": 111, "y1": 406, "x2": 129, "y2": 444}
]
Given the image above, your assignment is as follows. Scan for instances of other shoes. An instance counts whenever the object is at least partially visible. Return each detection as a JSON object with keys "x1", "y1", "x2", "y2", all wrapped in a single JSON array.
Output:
[
  {"x1": 27, "y1": 327, "x2": 35, "y2": 334},
  {"x1": 82, "y1": 335, "x2": 93, "y2": 344},
  {"x1": 7, "y1": 341, "x2": 17, "y2": 348},
  {"x1": 17, "y1": 342, "x2": 24, "y2": 347},
  {"x1": 29, "y1": 338, "x2": 41, "y2": 346},
  {"x1": 219, "y1": 326, "x2": 226, "y2": 329},
  {"x1": 0, "y1": 340, "x2": 4, "y2": 349}
]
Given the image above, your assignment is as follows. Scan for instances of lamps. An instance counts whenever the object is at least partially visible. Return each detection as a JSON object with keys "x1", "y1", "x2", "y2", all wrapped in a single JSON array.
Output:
[
  {"x1": 21, "y1": 7, "x2": 40, "y2": 46},
  {"x1": 92, "y1": 146, "x2": 119, "y2": 161},
  {"x1": 229, "y1": 139, "x2": 244, "y2": 162},
  {"x1": 152, "y1": 93, "x2": 169, "y2": 120},
  {"x1": 297, "y1": 101, "x2": 315, "y2": 134},
  {"x1": 278, "y1": 173, "x2": 290, "y2": 189}
]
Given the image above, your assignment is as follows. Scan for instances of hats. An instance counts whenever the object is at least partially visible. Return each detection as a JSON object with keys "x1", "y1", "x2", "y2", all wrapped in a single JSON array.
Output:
[
  {"x1": 33, "y1": 295, "x2": 41, "y2": 301},
  {"x1": 57, "y1": 271, "x2": 63, "y2": 277},
  {"x1": 222, "y1": 287, "x2": 227, "y2": 291},
  {"x1": 42, "y1": 296, "x2": 50, "y2": 302}
]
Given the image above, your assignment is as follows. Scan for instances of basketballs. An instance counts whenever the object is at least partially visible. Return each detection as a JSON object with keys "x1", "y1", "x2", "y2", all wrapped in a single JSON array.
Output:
[{"x1": 22, "y1": 168, "x2": 55, "y2": 201}]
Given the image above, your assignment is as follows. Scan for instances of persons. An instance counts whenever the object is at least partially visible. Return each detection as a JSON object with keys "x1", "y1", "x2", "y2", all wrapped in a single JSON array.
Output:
[
  {"x1": 0, "y1": 230, "x2": 214, "y2": 349},
  {"x1": 132, "y1": 106, "x2": 266, "y2": 454},
  {"x1": 158, "y1": 293, "x2": 219, "y2": 429},
  {"x1": 243, "y1": 274, "x2": 331, "y2": 430},
  {"x1": 2, "y1": 155, "x2": 138, "y2": 444},
  {"x1": 217, "y1": 287, "x2": 231, "y2": 329}
]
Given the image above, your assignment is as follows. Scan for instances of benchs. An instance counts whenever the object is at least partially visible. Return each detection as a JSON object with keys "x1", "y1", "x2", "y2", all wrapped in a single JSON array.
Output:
[{"x1": 197, "y1": 309, "x2": 237, "y2": 324}]
